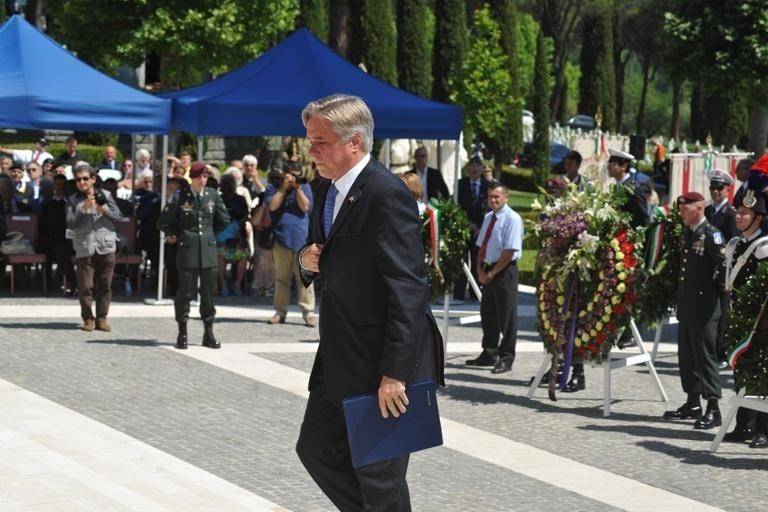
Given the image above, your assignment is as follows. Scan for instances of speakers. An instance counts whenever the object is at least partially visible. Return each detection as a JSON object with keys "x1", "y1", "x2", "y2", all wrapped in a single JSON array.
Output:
[{"x1": 629, "y1": 135, "x2": 646, "y2": 161}]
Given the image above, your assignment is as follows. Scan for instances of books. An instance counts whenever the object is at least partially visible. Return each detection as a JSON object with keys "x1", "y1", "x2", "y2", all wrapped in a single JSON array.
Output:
[{"x1": 341, "y1": 377, "x2": 443, "y2": 469}]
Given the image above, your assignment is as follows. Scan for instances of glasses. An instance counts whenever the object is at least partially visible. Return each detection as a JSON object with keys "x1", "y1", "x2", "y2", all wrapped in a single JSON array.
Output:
[
  {"x1": 123, "y1": 165, "x2": 132, "y2": 168},
  {"x1": 27, "y1": 168, "x2": 36, "y2": 173},
  {"x1": 76, "y1": 176, "x2": 89, "y2": 182}
]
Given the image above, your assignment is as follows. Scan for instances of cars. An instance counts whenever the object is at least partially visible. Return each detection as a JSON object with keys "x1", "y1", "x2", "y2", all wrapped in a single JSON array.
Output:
[
  {"x1": 570, "y1": 114, "x2": 594, "y2": 128},
  {"x1": 501, "y1": 139, "x2": 572, "y2": 181},
  {"x1": 521, "y1": 109, "x2": 534, "y2": 125}
]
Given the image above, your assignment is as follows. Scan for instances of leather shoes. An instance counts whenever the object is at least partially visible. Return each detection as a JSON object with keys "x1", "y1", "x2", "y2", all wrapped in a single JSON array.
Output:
[
  {"x1": 177, "y1": 331, "x2": 188, "y2": 349},
  {"x1": 465, "y1": 348, "x2": 585, "y2": 393},
  {"x1": 203, "y1": 327, "x2": 220, "y2": 348},
  {"x1": 663, "y1": 403, "x2": 768, "y2": 449}
]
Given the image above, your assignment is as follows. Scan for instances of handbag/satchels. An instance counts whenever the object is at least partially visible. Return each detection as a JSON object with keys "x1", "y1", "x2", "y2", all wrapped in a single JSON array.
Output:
[{"x1": 1, "y1": 239, "x2": 37, "y2": 256}]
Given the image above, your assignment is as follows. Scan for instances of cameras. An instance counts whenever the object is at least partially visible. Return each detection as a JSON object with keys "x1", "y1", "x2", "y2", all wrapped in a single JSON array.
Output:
[
  {"x1": 92, "y1": 188, "x2": 107, "y2": 206},
  {"x1": 292, "y1": 174, "x2": 307, "y2": 185}
]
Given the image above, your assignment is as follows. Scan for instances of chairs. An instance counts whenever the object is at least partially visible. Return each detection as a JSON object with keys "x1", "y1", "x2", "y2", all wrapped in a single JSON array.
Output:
[
  {"x1": 6, "y1": 214, "x2": 50, "y2": 294},
  {"x1": 164, "y1": 257, "x2": 255, "y2": 301},
  {"x1": 61, "y1": 256, "x2": 76, "y2": 295},
  {"x1": 114, "y1": 218, "x2": 141, "y2": 295}
]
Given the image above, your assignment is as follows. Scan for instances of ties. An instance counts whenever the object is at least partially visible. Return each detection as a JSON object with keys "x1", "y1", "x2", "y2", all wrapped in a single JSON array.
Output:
[
  {"x1": 471, "y1": 178, "x2": 478, "y2": 206},
  {"x1": 323, "y1": 182, "x2": 339, "y2": 240},
  {"x1": 479, "y1": 213, "x2": 497, "y2": 261}
]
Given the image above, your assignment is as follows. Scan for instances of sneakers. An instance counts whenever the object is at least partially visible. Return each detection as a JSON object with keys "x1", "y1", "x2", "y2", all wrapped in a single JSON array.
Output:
[
  {"x1": 81, "y1": 319, "x2": 94, "y2": 332},
  {"x1": 303, "y1": 311, "x2": 316, "y2": 327},
  {"x1": 95, "y1": 319, "x2": 112, "y2": 332},
  {"x1": 219, "y1": 288, "x2": 229, "y2": 297},
  {"x1": 268, "y1": 312, "x2": 286, "y2": 324},
  {"x1": 233, "y1": 289, "x2": 243, "y2": 296}
]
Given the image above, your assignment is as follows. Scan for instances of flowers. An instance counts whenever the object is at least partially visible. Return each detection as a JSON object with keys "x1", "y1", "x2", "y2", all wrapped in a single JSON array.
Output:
[{"x1": 521, "y1": 174, "x2": 687, "y2": 365}]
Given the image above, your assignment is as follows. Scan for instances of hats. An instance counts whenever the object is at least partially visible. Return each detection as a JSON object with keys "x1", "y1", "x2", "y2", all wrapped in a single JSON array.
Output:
[
  {"x1": 189, "y1": 161, "x2": 214, "y2": 179},
  {"x1": 607, "y1": 147, "x2": 634, "y2": 163},
  {"x1": 562, "y1": 151, "x2": 582, "y2": 161},
  {"x1": 8, "y1": 162, "x2": 24, "y2": 172},
  {"x1": 676, "y1": 168, "x2": 767, "y2": 216}
]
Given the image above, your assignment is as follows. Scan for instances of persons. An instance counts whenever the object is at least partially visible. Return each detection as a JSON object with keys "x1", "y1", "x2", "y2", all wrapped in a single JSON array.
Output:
[
  {"x1": 605, "y1": 132, "x2": 765, "y2": 350},
  {"x1": 404, "y1": 144, "x2": 449, "y2": 209},
  {"x1": 453, "y1": 155, "x2": 488, "y2": 302},
  {"x1": 399, "y1": 171, "x2": 426, "y2": 219},
  {"x1": 664, "y1": 192, "x2": 728, "y2": 431},
  {"x1": 295, "y1": 92, "x2": 446, "y2": 511},
  {"x1": 156, "y1": 162, "x2": 224, "y2": 349},
  {"x1": 541, "y1": 174, "x2": 586, "y2": 393},
  {"x1": 465, "y1": 181, "x2": 525, "y2": 375},
  {"x1": 262, "y1": 159, "x2": 319, "y2": 330},
  {"x1": 65, "y1": 161, "x2": 122, "y2": 333},
  {"x1": 1, "y1": 134, "x2": 322, "y2": 302},
  {"x1": 723, "y1": 188, "x2": 768, "y2": 450},
  {"x1": 564, "y1": 151, "x2": 588, "y2": 190},
  {"x1": 484, "y1": 162, "x2": 500, "y2": 190}
]
